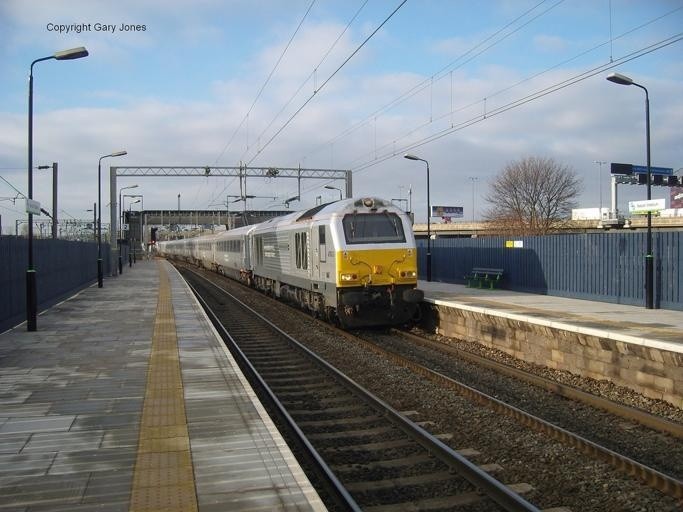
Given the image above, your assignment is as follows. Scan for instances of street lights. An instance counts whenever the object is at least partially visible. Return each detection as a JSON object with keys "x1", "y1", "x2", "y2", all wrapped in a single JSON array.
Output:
[
  {"x1": 20, "y1": 43, "x2": 91, "y2": 334},
  {"x1": 321, "y1": 183, "x2": 343, "y2": 200},
  {"x1": 94, "y1": 150, "x2": 142, "y2": 292},
  {"x1": 401, "y1": 150, "x2": 435, "y2": 282},
  {"x1": 602, "y1": 70, "x2": 657, "y2": 311},
  {"x1": 465, "y1": 176, "x2": 479, "y2": 223},
  {"x1": 591, "y1": 159, "x2": 607, "y2": 223}
]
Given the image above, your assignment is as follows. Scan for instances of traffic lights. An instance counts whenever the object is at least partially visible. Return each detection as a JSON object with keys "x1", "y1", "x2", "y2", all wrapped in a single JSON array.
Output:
[{"x1": 149, "y1": 226, "x2": 158, "y2": 245}]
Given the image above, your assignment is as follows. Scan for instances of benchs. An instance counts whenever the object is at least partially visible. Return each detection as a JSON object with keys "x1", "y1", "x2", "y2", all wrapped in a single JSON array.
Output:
[{"x1": 464, "y1": 267, "x2": 504, "y2": 290}]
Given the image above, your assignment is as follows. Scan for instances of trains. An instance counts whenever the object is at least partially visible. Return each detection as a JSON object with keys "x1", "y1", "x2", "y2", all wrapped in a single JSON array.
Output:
[{"x1": 147, "y1": 194, "x2": 428, "y2": 333}]
